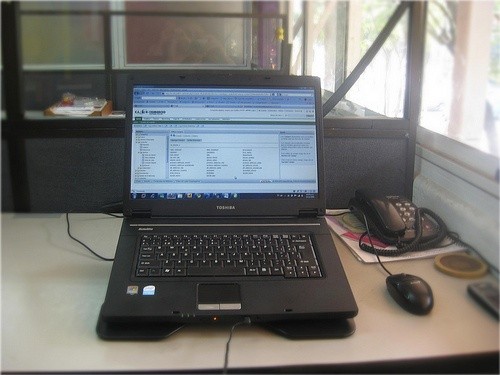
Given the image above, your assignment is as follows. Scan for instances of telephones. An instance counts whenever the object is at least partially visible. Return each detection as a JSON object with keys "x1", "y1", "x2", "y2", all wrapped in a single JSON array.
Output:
[{"x1": 347, "y1": 190, "x2": 439, "y2": 245}]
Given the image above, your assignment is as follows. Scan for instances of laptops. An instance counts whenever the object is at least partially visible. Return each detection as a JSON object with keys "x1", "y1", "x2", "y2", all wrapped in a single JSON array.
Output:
[{"x1": 95, "y1": 71, "x2": 357, "y2": 324}]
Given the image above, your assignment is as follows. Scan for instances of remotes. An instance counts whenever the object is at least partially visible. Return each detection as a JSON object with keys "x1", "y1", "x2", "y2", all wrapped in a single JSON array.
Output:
[{"x1": 468, "y1": 279, "x2": 499, "y2": 320}]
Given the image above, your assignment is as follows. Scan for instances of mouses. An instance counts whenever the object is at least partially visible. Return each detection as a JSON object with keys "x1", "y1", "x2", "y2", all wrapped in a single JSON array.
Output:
[{"x1": 386, "y1": 272, "x2": 434, "y2": 315}]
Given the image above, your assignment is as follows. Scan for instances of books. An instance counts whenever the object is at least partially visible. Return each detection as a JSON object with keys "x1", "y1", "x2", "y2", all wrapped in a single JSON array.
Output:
[{"x1": 324, "y1": 207, "x2": 470, "y2": 265}]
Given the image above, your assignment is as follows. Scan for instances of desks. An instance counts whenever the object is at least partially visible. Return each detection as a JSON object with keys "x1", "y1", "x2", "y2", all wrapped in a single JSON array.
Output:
[{"x1": 1, "y1": 211, "x2": 500, "y2": 375}]
